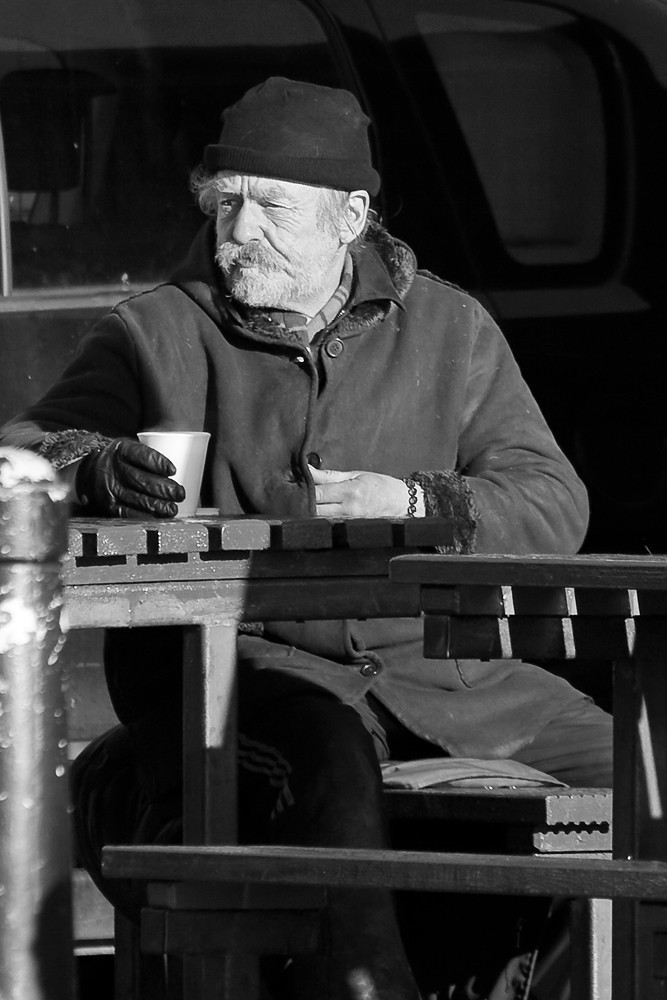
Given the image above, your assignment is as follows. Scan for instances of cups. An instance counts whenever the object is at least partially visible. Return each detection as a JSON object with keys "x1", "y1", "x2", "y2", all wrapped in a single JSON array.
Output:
[{"x1": 136, "y1": 431, "x2": 211, "y2": 520}]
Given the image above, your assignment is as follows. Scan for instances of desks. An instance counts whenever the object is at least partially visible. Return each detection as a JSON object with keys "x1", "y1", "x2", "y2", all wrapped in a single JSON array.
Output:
[
  {"x1": 402, "y1": 555, "x2": 666, "y2": 997},
  {"x1": 52, "y1": 514, "x2": 461, "y2": 843}
]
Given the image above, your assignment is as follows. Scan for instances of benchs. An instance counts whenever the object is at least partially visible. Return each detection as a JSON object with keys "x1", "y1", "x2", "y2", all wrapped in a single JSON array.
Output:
[
  {"x1": 379, "y1": 784, "x2": 612, "y2": 997},
  {"x1": 98, "y1": 842, "x2": 666, "y2": 999}
]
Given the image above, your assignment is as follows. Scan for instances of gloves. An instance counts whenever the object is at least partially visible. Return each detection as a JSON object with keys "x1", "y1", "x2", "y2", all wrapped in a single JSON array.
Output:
[{"x1": 78, "y1": 436, "x2": 186, "y2": 520}]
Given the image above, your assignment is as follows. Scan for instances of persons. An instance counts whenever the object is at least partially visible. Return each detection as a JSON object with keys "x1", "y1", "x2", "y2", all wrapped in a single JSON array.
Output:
[{"x1": 0, "y1": 75, "x2": 667, "y2": 1000}]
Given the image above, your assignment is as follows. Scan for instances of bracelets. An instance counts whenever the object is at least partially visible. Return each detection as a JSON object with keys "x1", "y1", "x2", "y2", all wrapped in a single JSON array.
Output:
[{"x1": 402, "y1": 478, "x2": 418, "y2": 518}]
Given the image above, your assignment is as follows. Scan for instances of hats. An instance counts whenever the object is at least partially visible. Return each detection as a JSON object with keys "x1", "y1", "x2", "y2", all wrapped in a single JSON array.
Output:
[{"x1": 208, "y1": 76, "x2": 383, "y2": 197}]
